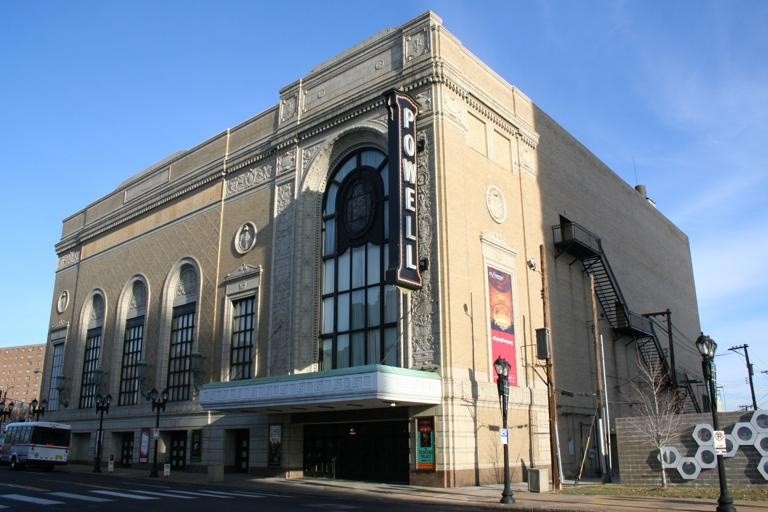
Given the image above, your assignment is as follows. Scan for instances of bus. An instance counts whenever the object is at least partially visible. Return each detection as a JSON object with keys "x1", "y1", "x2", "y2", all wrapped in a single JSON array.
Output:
[{"x1": 0, "y1": 420, "x2": 73, "y2": 473}]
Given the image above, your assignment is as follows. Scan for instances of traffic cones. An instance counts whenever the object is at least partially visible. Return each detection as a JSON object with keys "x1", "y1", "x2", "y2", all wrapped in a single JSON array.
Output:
[
  {"x1": 492, "y1": 357, "x2": 516, "y2": 505},
  {"x1": 0, "y1": 400, "x2": 14, "y2": 425},
  {"x1": 695, "y1": 331, "x2": 737, "y2": 511},
  {"x1": 31, "y1": 398, "x2": 48, "y2": 421},
  {"x1": 91, "y1": 394, "x2": 114, "y2": 472},
  {"x1": 149, "y1": 388, "x2": 170, "y2": 477}
]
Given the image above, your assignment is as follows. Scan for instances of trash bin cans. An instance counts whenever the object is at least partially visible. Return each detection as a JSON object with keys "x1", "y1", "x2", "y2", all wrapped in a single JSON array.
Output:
[{"x1": 528, "y1": 468, "x2": 549, "y2": 493}]
